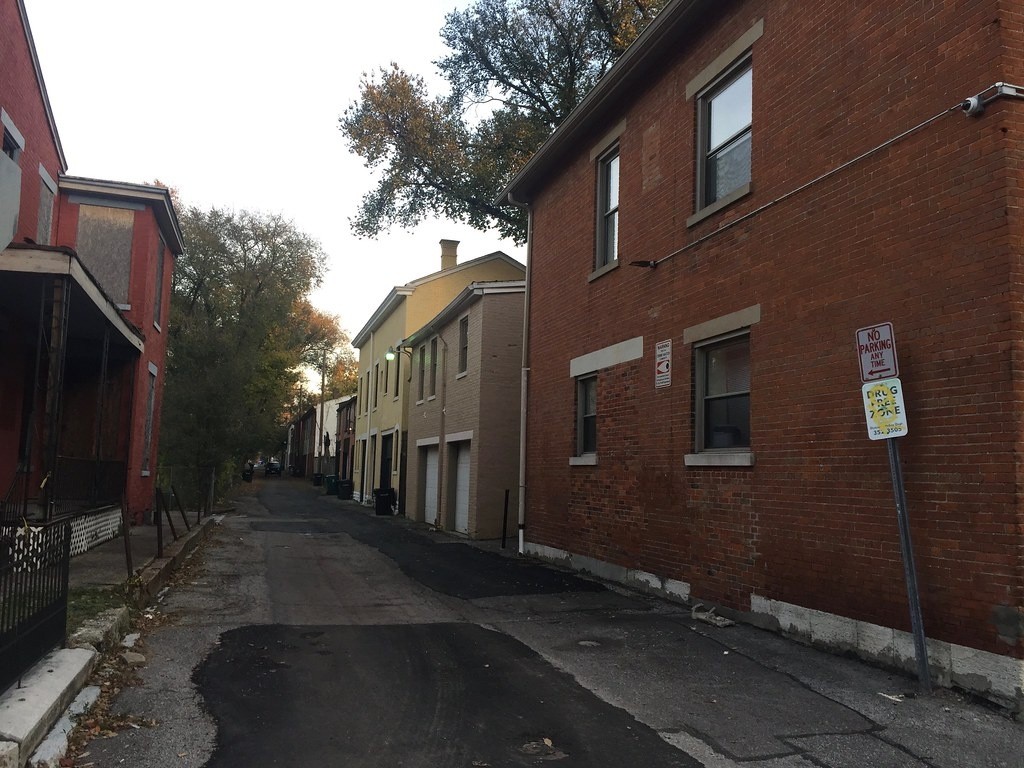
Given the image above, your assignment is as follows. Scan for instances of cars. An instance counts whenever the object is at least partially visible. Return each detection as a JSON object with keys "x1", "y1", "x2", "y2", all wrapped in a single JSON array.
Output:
[
  {"x1": 264, "y1": 461, "x2": 281, "y2": 476},
  {"x1": 242, "y1": 462, "x2": 254, "y2": 483}
]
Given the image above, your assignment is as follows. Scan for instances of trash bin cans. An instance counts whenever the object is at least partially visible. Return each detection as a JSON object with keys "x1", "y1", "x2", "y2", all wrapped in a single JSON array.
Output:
[
  {"x1": 325, "y1": 475, "x2": 339, "y2": 495},
  {"x1": 336, "y1": 478, "x2": 354, "y2": 500},
  {"x1": 244, "y1": 468, "x2": 252, "y2": 482},
  {"x1": 373, "y1": 488, "x2": 396, "y2": 516},
  {"x1": 312, "y1": 473, "x2": 323, "y2": 486}
]
